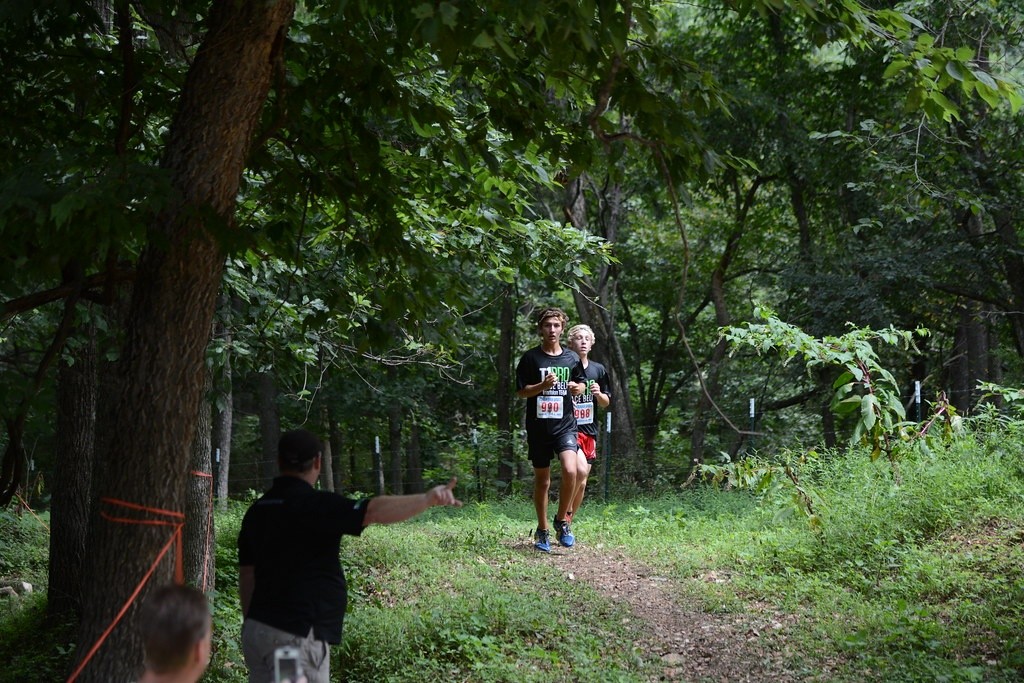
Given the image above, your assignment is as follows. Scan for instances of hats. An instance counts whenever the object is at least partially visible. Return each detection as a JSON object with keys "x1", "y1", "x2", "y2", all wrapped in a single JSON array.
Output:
[{"x1": 277, "y1": 430, "x2": 323, "y2": 463}]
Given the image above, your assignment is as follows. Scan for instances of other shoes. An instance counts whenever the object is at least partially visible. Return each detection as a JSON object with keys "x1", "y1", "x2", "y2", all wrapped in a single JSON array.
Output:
[{"x1": 566, "y1": 513, "x2": 572, "y2": 526}]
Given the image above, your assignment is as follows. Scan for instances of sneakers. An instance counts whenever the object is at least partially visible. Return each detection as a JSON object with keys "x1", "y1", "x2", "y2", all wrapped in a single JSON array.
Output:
[
  {"x1": 553, "y1": 515, "x2": 574, "y2": 547},
  {"x1": 534, "y1": 527, "x2": 551, "y2": 553}
]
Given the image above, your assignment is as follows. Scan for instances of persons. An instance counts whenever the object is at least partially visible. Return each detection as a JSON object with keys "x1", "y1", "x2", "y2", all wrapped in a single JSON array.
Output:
[
  {"x1": 237, "y1": 430, "x2": 464, "y2": 683},
  {"x1": 515, "y1": 308, "x2": 588, "y2": 553},
  {"x1": 135, "y1": 583, "x2": 214, "y2": 683},
  {"x1": 566, "y1": 322, "x2": 612, "y2": 526}
]
